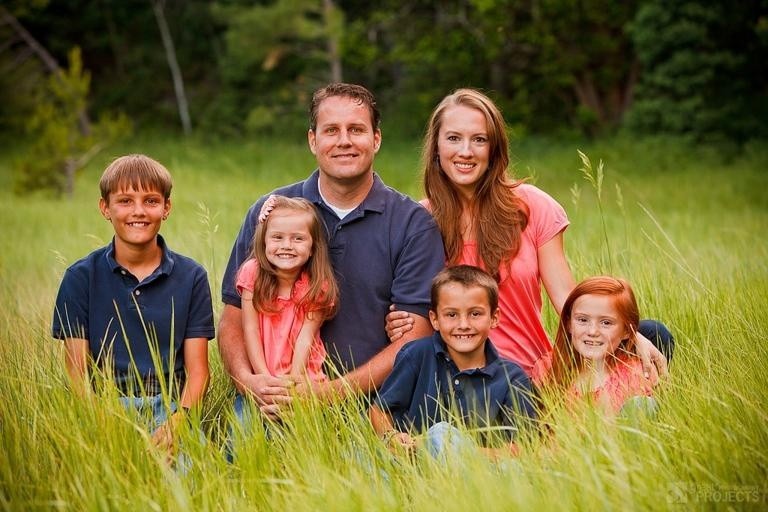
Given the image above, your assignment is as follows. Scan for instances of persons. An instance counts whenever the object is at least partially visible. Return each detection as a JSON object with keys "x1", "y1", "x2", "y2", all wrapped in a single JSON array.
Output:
[
  {"x1": 384, "y1": 87, "x2": 675, "y2": 392},
  {"x1": 215, "y1": 80, "x2": 447, "y2": 464},
  {"x1": 52, "y1": 151, "x2": 228, "y2": 470},
  {"x1": 372, "y1": 262, "x2": 538, "y2": 467},
  {"x1": 234, "y1": 192, "x2": 341, "y2": 386},
  {"x1": 532, "y1": 276, "x2": 658, "y2": 445}
]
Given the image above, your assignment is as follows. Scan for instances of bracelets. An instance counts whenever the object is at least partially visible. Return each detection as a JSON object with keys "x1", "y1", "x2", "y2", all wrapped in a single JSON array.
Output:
[
  {"x1": 381, "y1": 429, "x2": 400, "y2": 445},
  {"x1": 172, "y1": 404, "x2": 198, "y2": 418}
]
